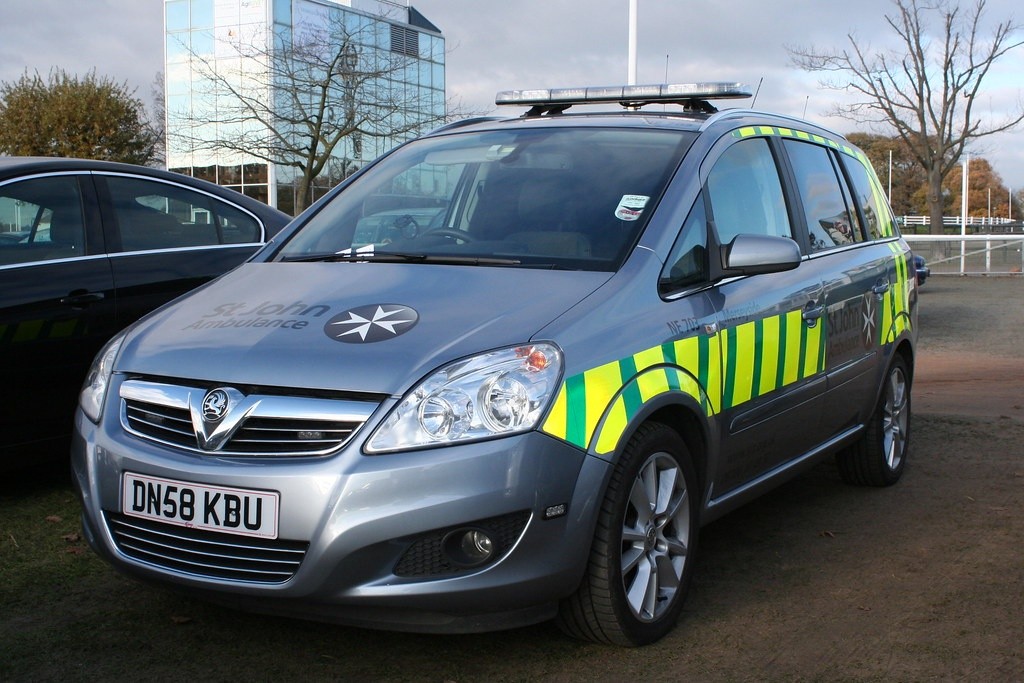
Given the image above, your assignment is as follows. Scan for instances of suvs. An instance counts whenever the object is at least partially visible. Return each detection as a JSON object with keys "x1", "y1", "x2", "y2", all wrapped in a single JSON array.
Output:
[{"x1": 72, "y1": 93, "x2": 918, "y2": 655}]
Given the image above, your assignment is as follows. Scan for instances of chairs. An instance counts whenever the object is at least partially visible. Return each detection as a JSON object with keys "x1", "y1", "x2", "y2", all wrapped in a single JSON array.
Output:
[
  {"x1": 48, "y1": 198, "x2": 80, "y2": 261},
  {"x1": 505, "y1": 177, "x2": 593, "y2": 256}
]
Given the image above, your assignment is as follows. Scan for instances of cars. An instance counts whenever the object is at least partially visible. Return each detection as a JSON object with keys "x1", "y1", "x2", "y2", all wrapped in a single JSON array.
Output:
[
  {"x1": 0, "y1": 154, "x2": 298, "y2": 476},
  {"x1": 352, "y1": 206, "x2": 446, "y2": 246}
]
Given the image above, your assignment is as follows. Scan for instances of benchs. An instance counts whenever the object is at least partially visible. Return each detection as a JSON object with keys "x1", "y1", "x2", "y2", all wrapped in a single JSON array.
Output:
[{"x1": 116, "y1": 207, "x2": 225, "y2": 250}]
